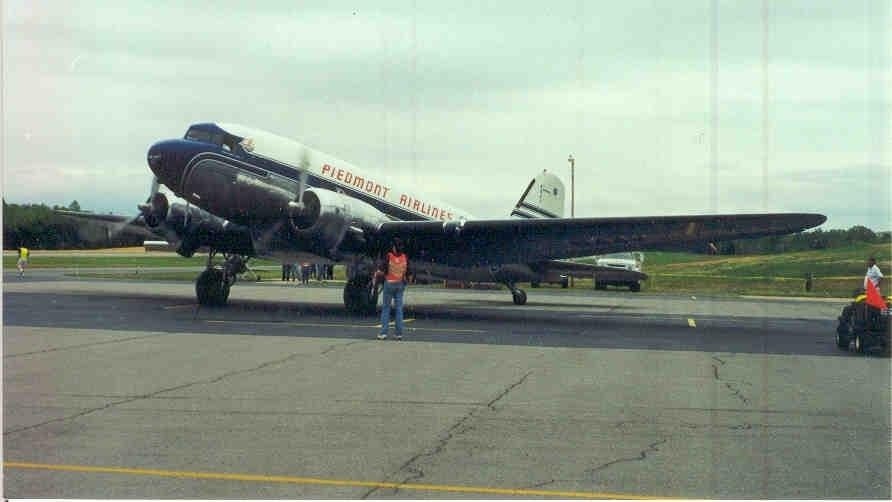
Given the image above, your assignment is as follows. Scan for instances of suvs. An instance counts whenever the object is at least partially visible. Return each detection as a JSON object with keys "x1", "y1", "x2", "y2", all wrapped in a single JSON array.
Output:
[
  {"x1": 528, "y1": 257, "x2": 571, "y2": 287},
  {"x1": 593, "y1": 250, "x2": 645, "y2": 291}
]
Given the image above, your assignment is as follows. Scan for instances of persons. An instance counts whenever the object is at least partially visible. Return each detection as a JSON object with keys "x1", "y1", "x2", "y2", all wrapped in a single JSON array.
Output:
[
  {"x1": 862, "y1": 256, "x2": 884, "y2": 294},
  {"x1": 16, "y1": 243, "x2": 30, "y2": 280},
  {"x1": 282, "y1": 264, "x2": 334, "y2": 284},
  {"x1": 374, "y1": 239, "x2": 410, "y2": 339}
]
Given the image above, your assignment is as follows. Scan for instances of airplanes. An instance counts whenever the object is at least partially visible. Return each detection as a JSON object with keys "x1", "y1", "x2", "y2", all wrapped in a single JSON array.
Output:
[{"x1": 51, "y1": 117, "x2": 826, "y2": 313}]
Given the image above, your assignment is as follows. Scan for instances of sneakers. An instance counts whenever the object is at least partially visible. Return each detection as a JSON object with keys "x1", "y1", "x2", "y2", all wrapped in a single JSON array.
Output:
[{"x1": 378, "y1": 333, "x2": 403, "y2": 341}]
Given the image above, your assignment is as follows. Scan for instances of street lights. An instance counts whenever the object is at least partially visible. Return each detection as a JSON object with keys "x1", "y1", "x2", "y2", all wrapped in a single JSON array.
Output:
[{"x1": 567, "y1": 153, "x2": 575, "y2": 220}]
